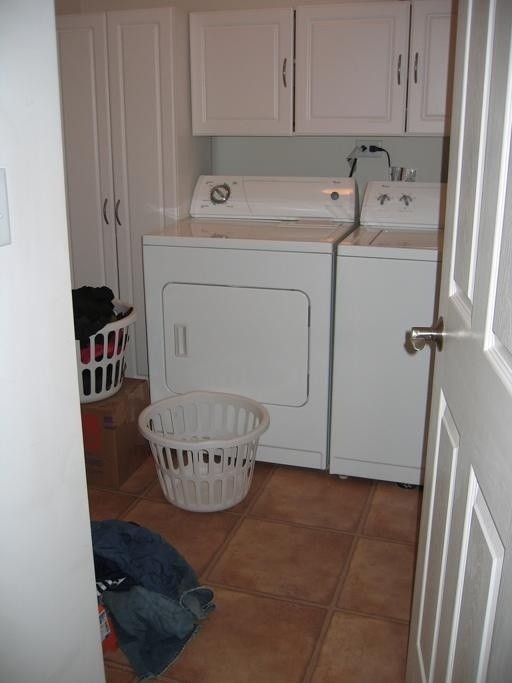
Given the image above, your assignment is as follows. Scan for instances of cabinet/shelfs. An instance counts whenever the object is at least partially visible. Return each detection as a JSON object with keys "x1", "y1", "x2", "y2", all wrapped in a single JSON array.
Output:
[
  {"x1": 57, "y1": 7, "x2": 213, "y2": 377},
  {"x1": 190, "y1": 7, "x2": 294, "y2": 136},
  {"x1": 292, "y1": 1, "x2": 410, "y2": 135},
  {"x1": 406, "y1": 1, "x2": 451, "y2": 137}
]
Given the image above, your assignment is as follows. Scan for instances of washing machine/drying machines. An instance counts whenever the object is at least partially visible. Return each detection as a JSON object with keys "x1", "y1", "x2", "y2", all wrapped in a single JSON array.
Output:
[
  {"x1": 328, "y1": 181, "x2": 442, "y2": 491},
  {"x1": 141, "y1": 175, "x2": 361, "y2": 474}
]
